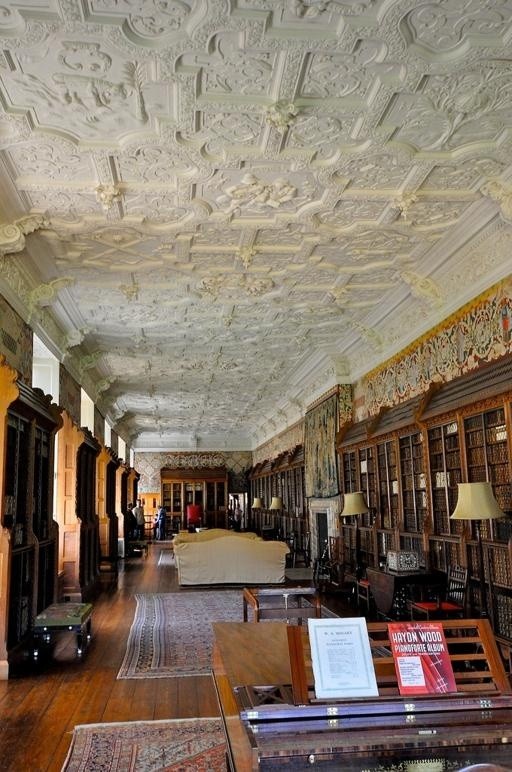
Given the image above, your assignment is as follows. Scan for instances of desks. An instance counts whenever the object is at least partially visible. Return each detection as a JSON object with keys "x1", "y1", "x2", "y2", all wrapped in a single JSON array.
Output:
[{"x1": 366, "y1": 565, "x2": 448, "y2": 616}]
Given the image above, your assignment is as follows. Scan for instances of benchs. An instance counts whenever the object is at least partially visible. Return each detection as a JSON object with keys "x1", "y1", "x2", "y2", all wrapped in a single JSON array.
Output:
[{"x1": 31, "y1": 601, "x2": 95, "y2": 657}]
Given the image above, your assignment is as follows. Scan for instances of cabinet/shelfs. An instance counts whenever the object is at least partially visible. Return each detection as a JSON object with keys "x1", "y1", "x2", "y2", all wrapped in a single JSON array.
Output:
[{"x1": 161, "y1": 479, "x2": 227, "y2": 530}]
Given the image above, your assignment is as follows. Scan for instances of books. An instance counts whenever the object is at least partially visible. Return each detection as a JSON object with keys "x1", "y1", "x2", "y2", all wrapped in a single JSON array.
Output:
[{"x1": 386, "y1": 622, "x2": 458, "y2": 697}]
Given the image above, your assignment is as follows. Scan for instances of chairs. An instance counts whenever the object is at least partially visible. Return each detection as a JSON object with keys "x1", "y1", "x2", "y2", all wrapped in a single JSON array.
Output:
[
  {"x1": 293, "y1": 531, "x2": 311, "y2": 568},
  {"x1": 409, "y1": 561, "x2": 468, "y2": 636}
]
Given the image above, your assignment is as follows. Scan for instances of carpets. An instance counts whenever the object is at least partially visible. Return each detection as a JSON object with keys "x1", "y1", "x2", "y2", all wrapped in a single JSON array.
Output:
[
  {"x1": 116, "y1": 588, "x2": 393, "y2": 680},
  {"x1": 59, "y1": 716, "x2": 229, "y2": 772}
]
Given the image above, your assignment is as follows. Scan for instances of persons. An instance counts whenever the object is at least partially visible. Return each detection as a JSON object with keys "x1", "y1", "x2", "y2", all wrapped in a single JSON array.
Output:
[
  {"x1": 132, "y1": 499, "x2": 148, "y2": 540},
  {"x1": 233, "y1": 503, "x2": 243, "y2": 532},
  {"x1": 127, "y1": 502, "x2": 138, "y2": 541},
  {"x1": 154, "y1": 505, "x2": 167, "y2": 540}
]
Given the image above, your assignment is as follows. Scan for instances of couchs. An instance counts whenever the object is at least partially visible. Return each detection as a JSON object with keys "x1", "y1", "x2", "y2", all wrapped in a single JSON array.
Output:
[{"x1": 172, "y1": 528, "x2": 291, "y2": 585}]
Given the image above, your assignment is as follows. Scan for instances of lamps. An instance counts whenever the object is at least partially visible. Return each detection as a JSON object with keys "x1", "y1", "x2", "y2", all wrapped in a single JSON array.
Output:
[
  {"x1": 450, "y1": 482, "x2": 506, "y2": 619},
  {"x1": 251, "y1": 497, "x2": 282, "y2": 528},
  {"x1": 340, "y1": 491, "x2": 370, "y2": 578}
]
